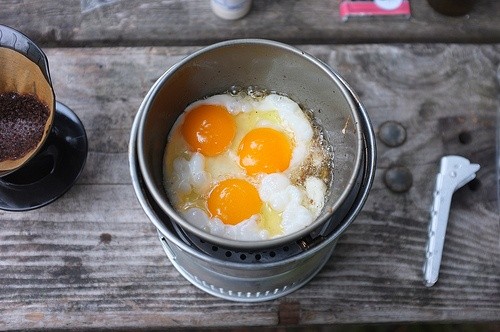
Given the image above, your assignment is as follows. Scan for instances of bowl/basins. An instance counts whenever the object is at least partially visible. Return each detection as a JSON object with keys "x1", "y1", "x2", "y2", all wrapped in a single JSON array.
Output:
[{"x1": 0, "y1": 23, "x2": 56, "y2": 178}]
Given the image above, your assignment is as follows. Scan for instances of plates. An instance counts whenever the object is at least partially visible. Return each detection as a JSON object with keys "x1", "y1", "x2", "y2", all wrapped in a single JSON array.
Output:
[{"x1": 0, "y1": 100, "x2": 89, "y2": 212}]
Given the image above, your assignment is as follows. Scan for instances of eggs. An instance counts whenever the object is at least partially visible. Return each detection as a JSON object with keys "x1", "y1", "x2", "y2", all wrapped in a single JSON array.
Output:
[{"x1": 161, "y1": 90, "x2": 328, "y2": 242}]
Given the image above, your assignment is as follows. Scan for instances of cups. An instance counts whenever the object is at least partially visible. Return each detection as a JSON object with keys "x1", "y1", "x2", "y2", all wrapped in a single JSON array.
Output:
[
  {"x1": 211, "y1": 0, "x2": 251, "y2": 20},
  {"x1": 128, "y1": 38, "x2": 377, "y2": 304}
]
